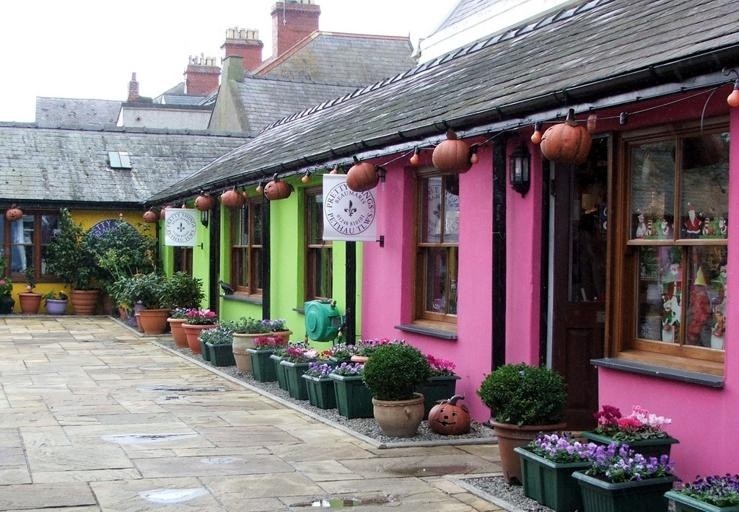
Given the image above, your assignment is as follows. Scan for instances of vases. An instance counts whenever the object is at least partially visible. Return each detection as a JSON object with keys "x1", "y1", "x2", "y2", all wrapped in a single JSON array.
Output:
[
  {"x1": 567, "y1": 465, "x2": 678, "y2": 512},
  {"x1": 516, "y1": 445, "x2": 589, "y2": 509},
  {"x1": 589, "y1": 427, "x2": 680, "y2": 465},
  {"x1": 665, "y1": 483, "x2": 722, "y2": 511}
]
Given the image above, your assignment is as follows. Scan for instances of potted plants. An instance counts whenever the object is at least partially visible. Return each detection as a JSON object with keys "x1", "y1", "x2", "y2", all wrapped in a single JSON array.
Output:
[
  {"x1": 476, "y1": 360, "x2": 573, "y2": 485},
  {"x1": 0, "y1": 205, "x2": 204, "y2": 333},
  {"x1": 363, "y1": 341, "x2": 428, "y2": 436}
]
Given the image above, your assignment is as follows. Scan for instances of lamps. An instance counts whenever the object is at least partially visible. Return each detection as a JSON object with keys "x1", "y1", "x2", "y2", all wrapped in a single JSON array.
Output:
[
  {"x1": 506, "y1": 137, "x2": 534, "y2": 198},
  {"x1": 200, "y1": 208, "x2": 208, "y2": 229}
]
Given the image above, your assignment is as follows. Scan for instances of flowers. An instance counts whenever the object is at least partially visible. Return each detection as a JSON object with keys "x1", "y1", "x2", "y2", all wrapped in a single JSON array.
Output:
[
  {"x1": 163, "y1": 300, "x2": 461, "y2": 420},
  {"x1": 596, "y1": 406, "x2": 672, "y2": 441},
  {"x1": 589, "y1": 444, "x2": 668, "y2": 476},
  {"x1": 682, "y1": 471, "x2": 737, "y2": 504},
  {"x1": 517, "y1": 432, "x2": 605, "y2": 460}
]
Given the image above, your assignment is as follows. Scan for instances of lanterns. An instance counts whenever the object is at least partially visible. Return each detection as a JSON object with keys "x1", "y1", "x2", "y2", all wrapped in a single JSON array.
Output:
[
  {"x1": 425, "y1": 402, "x2": 472, "y2": 437},
  {"x1": 431, "y1": 139, "x2": 473, "y2": 175},
  {"x1": 539, "y1": 122, "x2": 594, "y2": 166},
  {"x1": 142, "y1": 180, "x2": 293, "y2": 222},
  {"x1": 6, "y1": 205, "x2": 24, "y2": 223},
  {"x1": 345, "y1": 160, "x2": 379, "y2": 192}
]
